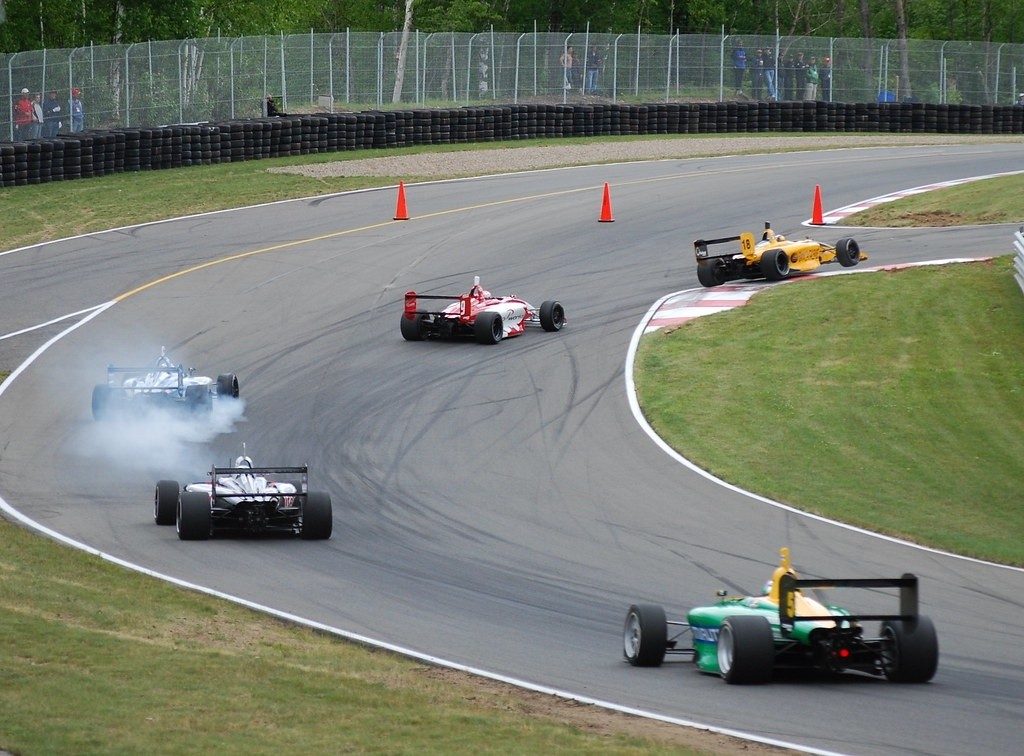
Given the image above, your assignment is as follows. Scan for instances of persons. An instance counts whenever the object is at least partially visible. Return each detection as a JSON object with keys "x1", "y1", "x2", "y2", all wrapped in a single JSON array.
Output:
[
  {"x1": 732, "y1": 39, "x2": 834, "y2": 101},
  {"x1": 13, "y1": 87, "x2": 84, "y2": 141},
  {"x1": 261, "y1": 95, "x2": 278, "y2": 117},
  {"x1": 558, "y1": 46, "x2": 602, "y2": 95}
]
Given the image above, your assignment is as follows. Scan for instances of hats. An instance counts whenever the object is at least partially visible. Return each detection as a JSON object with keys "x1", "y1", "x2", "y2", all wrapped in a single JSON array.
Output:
[
  {"x1": 824, "y1": 58, "x2": 830, "y2": 62},
  {"x1": 72, "y1": 89, "x2": 80, "y2": 94},
  {"x1": 21, "y1": 88, "x2": 29, "y2": 94},
  {"x1": 798, "y1": 53, "x2": 803, "y2": 57},
  {"x1": 789, "y1": 54, "x2": 794, "y2": 58},
  {"x1": 757, "y1": 50, "x2": 762, "y2": 53},
  {"x1": 811, "y1": 56, "x2": 814, "y2": 59}
]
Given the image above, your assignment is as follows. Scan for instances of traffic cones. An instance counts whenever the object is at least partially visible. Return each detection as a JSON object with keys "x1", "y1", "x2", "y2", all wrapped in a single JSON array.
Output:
[
  {"x1": 598, "y1": 181, "x2": 615, "y2": 222},
  {"x1": 392, "y1": 180, "x2": 410, "y2": 220},
  {"x1": 807, "y1": 184, "x2": 827, "y2": 227}
]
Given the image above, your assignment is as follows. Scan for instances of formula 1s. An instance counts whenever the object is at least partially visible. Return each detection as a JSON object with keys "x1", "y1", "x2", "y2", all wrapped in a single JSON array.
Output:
[
  {"x1": 400, "y1": 275, "x2": 568, "y2": 343},
  {"x1": 153, "y1": 444, "x2": 335, "y2": 539},
  {"x1": 692, "y1": 219, "x2": 868, "y2": 289},
  {"x1": 622, "y1": 549, "x2": 939, "y2": 687},
  {"x1": 91, "y1": 344, "x2": 242, "y2": 420}
]
